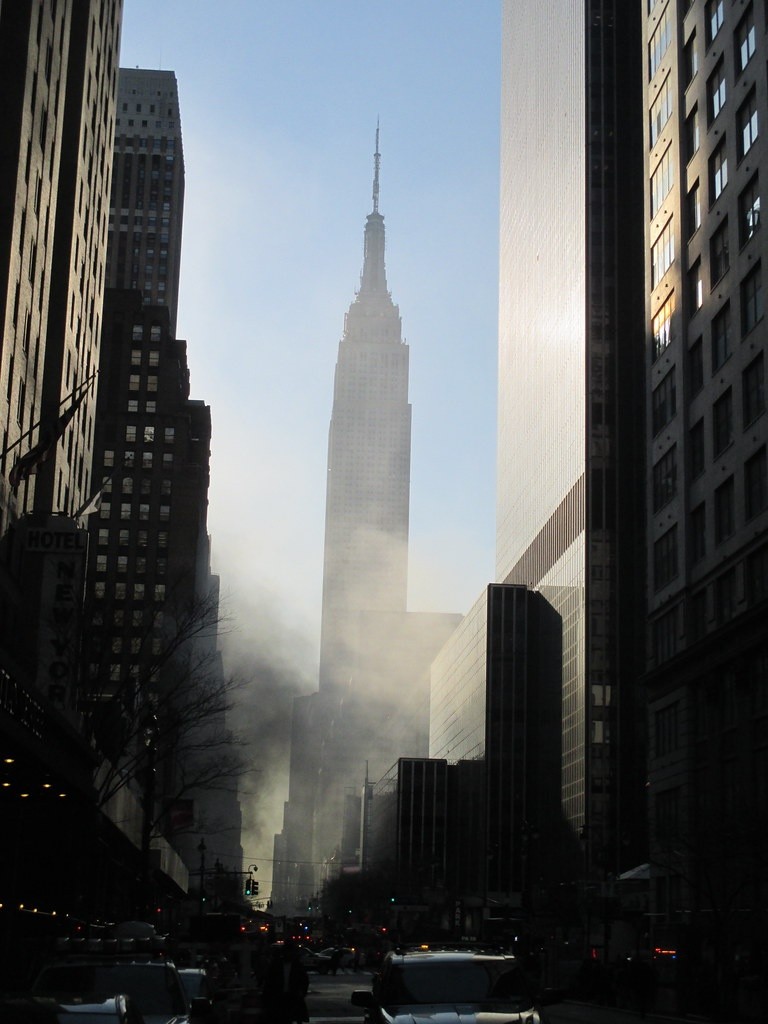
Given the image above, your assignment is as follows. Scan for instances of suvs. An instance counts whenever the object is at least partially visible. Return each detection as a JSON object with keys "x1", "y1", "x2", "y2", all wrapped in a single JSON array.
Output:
[{"x1": 351, "y1": 941, "x2": 566, "y2": 1024}]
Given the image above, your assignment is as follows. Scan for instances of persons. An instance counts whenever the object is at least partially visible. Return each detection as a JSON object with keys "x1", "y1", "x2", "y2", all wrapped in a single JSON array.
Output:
[
  {"x1": 329, "y1": 944, "x2": 344, "y2": 976},
  {"x1": 353, "y1": 945, "x2": 361, "y2": 972}
]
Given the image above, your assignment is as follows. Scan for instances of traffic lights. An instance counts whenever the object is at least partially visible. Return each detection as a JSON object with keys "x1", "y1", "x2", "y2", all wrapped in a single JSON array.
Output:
[
  {"x1": 251, "y1": 880, "x2": 258, "y2": 895},
  {"x1": 245, "y1": 880, "x2": 251, "y2": 895}
]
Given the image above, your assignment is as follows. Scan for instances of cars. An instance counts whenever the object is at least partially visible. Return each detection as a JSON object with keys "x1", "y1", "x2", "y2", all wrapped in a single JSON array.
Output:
[
  {"x1": 289, "y1": 944, "x2": 367, "y2": 976},
  {"x1": 0, "y1": 927, "x2": 211, "y2": 1024}
]
